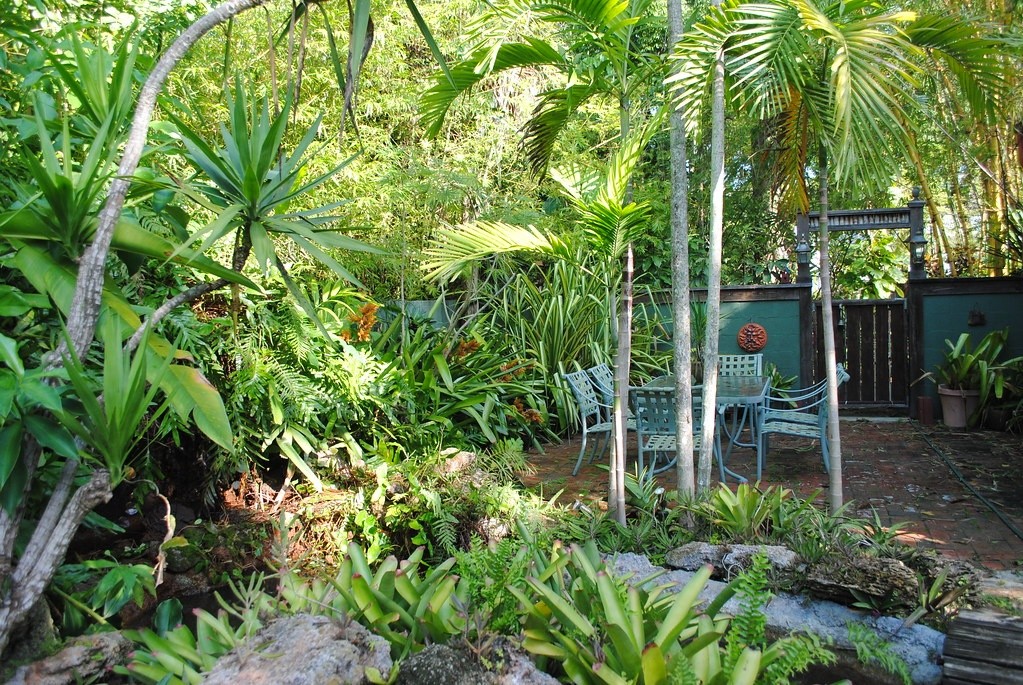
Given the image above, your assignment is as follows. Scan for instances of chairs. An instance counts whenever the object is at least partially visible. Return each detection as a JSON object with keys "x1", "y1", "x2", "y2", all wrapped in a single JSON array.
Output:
[
  {"x1": 562, "y1": 370, "x2": 656, "y2": 476},
  {"x1": 587, "y1": 363, "x2": 664, "y2": 460},
  {"x1": 629, "y1": 385, "x2": 725, "y2": 488},
  {"x1": 718, "y1": 353, "x2": 762, "y2": 440},
  {"x1": 762, "y1": 369, "x2": 851, "y2": 476},
  {"x1": 764, "y1": 363, "x2": 844, "y2": 452}
]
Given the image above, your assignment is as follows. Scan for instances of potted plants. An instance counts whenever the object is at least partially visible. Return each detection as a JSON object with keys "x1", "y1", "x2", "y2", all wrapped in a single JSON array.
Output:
[{"x1": 910, "y1": 325, "x2": 1023, "y2": 429}]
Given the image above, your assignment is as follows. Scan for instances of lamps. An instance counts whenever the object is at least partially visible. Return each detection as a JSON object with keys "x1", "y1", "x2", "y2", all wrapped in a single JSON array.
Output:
[
  {"x1": 795, "y1": 232, "x2": 812, "y2": 264},
  {"x1": 910, "y1": 226, "x2": 928, "y2": 264}
]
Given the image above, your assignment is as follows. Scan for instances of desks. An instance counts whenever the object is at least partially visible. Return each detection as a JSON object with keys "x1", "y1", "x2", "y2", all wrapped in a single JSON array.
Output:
[{"x1": 640, "y1": 375, "x2": 770, "y2": 482}]
